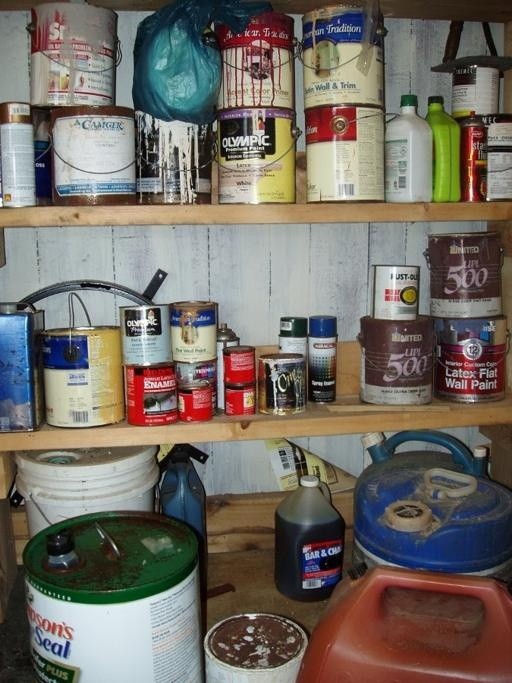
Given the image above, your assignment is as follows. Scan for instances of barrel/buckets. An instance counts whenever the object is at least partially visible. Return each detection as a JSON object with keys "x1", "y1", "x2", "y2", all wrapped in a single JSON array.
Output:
[
  {"x1": 302, "y1": 102, "x2": 386, "y2": 203},
  {"x1": 298, "y1": 4, "x2": 390, "y2": 112},
  {"x1": 360, "y1": 315, "x2": 433, "y2": 407},
  {"x1": 212, "y1": 9, "x2": 300, "y2": 112},
  {"x1": 434, "y1": 316, "x2": 512, "y2": 402},
  {"x1": 23, "y1": 511, "x2": 203, "y2": 683},
  {"x1": 448, "y1": 55, "x2": 503, "y2": 114},
  {"x1": 25, "y1": 2, "x2": 128, "y2": 106},
  {"x1": 203, "y1": 612, "x2": 307, "y2": 683},
  {"x1": 45, "y1": 104, "x2": 139, "y2": 204},
  {"x1": 160, "y1": 453, "x2": 208, "y2": 533},
  {"x1": 131, "y1": 104, "x2": 213, "y2": 206},
  {"x1": 275, "y1": 475, "x2": 345, "y2": 603},
  {"x1": 485, "y1": 113, "x2": 511, "y2": 202},
  {"x1": 40, "y1": 292, "x2": 309, "y2": 430},
  {"x1": 423, "y1": 230, "x2": 501, "y2": 319},
  {"x1": 214, "y1": 103, "x2": 303, "y2": 205},
  {"x1": 13, "y1": 451, "x2": 163, "y2": 538}
]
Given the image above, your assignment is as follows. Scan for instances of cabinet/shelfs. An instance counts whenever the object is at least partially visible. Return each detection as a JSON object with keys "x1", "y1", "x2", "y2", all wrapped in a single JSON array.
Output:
[{"x1": 0, "y1": 1, "x2": 512, "y2": 497}]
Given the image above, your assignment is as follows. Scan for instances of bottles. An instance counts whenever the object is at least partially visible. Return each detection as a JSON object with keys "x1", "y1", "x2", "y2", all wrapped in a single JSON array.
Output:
[
  {"x1": 277, "y1": 314, "x2": 339, "y2": 404},
  {"x1": 0, "y1": 98, "x2": 54, "y2": 209},
  {"x1": 383, "y1": 93, "x2": 491, "y2": 202}
]
